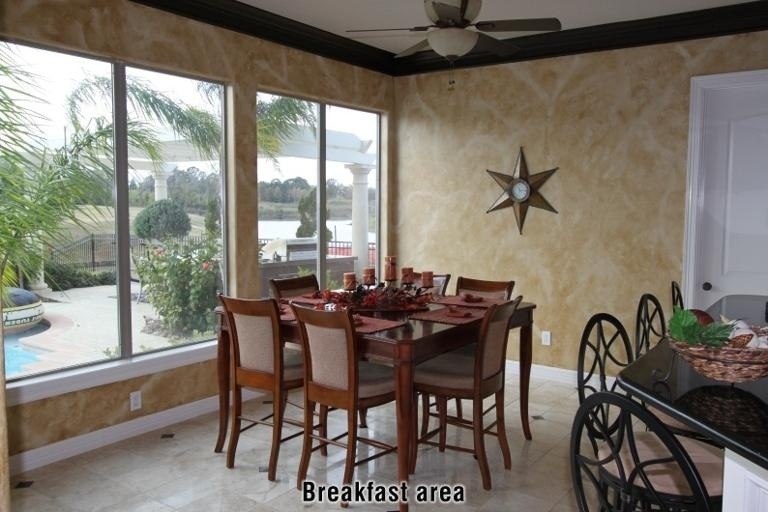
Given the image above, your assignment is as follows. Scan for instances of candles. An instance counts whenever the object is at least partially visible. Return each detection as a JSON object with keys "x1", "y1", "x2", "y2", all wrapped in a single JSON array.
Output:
[
  {"x1": 385, "y1": 256, "x2": 396, "y2": 282},
  {"x1": 343, "y1": 273, "x2": 355, "y2": 289},
  {"x1": 422, "y1": 272, "x2": 434, "y2": 287},
  {"x1": 363, "y1": 269, "x2": 375, "y2": 286},
  {"x1": 402, "y1": 268, "x2": 414, "y2": 284}
]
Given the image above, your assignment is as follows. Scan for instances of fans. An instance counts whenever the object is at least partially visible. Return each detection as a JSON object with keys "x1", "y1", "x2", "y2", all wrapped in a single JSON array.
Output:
[{"x1": 345, "y1": 0, "x2": 562, "y2": 59}]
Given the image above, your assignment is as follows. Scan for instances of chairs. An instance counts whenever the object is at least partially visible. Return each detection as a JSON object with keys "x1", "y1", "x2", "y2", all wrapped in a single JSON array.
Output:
[
  {"x1": 672, "y1": 280, "x2": 684, "y2": 315},
  {"x1": 408, "y1": 295, "x2": 523, "y2": 491},
  {"x1": 268, "y1": 274, "x2": 368, "y2": 427},
  {"x1": 400, "y1": 273, "x2": 451, "y2": 411},
  {"x1": 217, "y1": 292, "x2": 328, "y2": 481},
  {"x1": 421, "y1": 276, "x2": 515, "y2": 459},
  {"x1": 635, "y1": 293, "x2": 724, "y2": 449},
  {"x1": 288, "y1": 299, "x2": 398, "y2": 508},
  {"x1": 577, "y1": 313, "x2": 724, "y2": 512},
  {"x1": 569, "y1": 391, "x2": 715, "y2": 512}
]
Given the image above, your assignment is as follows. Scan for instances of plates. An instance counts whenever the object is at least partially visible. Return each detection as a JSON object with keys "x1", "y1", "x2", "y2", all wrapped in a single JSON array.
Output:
[
  {"x1": 462, "y1": 297, "x2": 484, "y2": 303},
  {"x1": 352, "y1": 318, "x2": 367, "y2": 325},
  {"x1": 445, "y1": 311, "x2": 469, "y2": 317}
]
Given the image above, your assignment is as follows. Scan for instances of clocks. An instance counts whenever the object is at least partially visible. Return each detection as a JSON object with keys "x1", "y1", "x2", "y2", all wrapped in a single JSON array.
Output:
[{"x1": 485, "y1": 145, "x2": 558, "y2": 234}]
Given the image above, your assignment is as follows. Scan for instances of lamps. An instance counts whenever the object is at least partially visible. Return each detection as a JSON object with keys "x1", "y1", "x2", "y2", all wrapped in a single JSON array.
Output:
[{"x1": 426, "y1": 28, "x2": 479, "y2": 91}]
